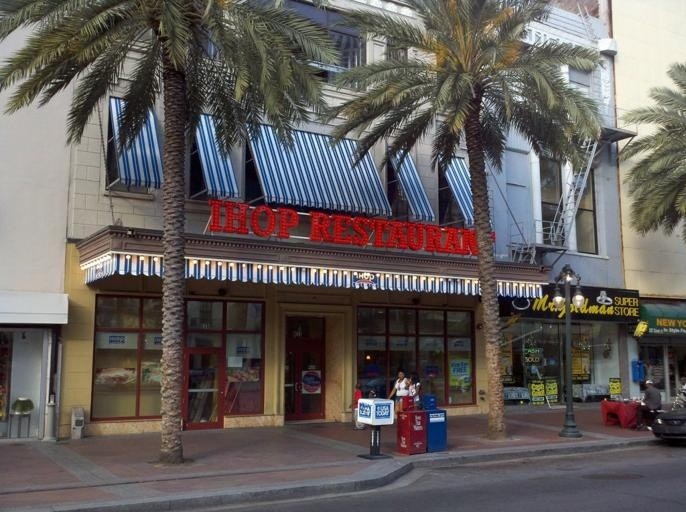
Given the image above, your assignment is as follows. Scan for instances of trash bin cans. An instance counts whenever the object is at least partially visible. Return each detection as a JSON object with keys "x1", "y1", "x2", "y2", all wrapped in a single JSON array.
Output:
[{"x1": 71, "y1": 407, "x2": 84, "y2": 440}]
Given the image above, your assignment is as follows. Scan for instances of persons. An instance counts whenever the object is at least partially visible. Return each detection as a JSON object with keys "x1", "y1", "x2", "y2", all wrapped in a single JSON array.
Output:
[
  {"x1": 405, "y1": 374, "x2": 422, "y2": 408},
  {"x1": 348, "y1": 383, "x2": 362, "y2": 431},
  {"x1": 387, "y1": 371, "x2": 410, "y2": 412},
  {"x1": 635, "y1": 378, "x2": 662, "y2": 429}
]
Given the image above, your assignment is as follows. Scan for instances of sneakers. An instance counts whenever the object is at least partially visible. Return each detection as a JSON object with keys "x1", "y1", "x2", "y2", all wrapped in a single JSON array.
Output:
[{"x1": 637, "y1": 424, "x2": 646, "y2": 430}]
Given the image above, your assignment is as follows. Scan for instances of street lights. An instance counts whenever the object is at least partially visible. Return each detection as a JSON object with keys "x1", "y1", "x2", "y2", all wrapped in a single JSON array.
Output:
[{"x1": 549, "y1": 260, "x2": 586, "y2": 440}]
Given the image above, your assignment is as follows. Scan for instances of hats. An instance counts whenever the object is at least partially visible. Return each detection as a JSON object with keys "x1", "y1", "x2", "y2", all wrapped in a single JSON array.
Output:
[{"x1": 646, "y1": 380, "x2": 653, "y2": 384}]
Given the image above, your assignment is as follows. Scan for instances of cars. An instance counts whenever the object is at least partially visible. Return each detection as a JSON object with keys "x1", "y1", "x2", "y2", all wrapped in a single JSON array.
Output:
[
  {"x1": 648, "y1": 383, "x2": 686, "y2": 447},
  {"x1": 360, "y1": 360, "x2": 474, "y2": 404}
]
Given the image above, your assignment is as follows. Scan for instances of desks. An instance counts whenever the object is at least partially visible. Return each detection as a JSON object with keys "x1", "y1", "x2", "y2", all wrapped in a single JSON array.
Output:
[{"x1": 602, "y1": 401, "x2": 641, "y2": 429}]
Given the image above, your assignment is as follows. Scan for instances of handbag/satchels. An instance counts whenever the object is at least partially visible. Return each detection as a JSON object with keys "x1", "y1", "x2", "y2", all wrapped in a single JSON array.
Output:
[{"x1": 419, "y1": 385, "x2": 424, "y2": 400}]
